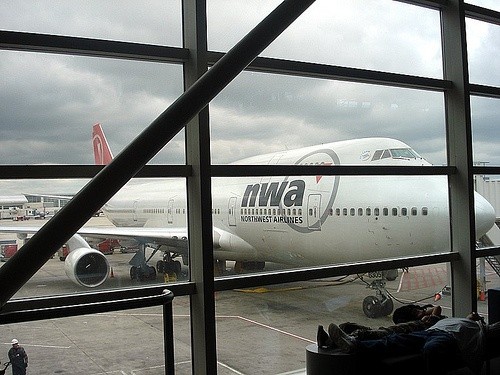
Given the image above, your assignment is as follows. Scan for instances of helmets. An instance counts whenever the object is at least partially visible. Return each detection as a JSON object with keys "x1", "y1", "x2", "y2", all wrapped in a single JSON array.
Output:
[{"x1": 11, "y1": 338, "x2": 18, "y2": 345}]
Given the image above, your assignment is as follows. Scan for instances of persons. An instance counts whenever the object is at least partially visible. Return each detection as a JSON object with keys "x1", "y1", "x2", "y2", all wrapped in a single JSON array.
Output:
[
  {"x1": 317, "y1": 304, "x2": 500, "y2": 375},
  {"x1": 8, "y1": 339, "x2": 28, "y2": 375}
]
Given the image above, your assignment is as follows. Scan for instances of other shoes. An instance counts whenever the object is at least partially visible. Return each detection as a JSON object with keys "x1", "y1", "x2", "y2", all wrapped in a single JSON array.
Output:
[
  {"x1": 316, "y1": 324, "x2": 330, "y2": 350},
  {"x1": 327, "y1": 322, "x2": 355, "y2": 353}
]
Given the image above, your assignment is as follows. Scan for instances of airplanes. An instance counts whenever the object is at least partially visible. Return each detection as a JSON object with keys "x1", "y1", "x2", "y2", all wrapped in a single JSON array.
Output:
[{"x1": 0, "y1": 123, "x2": 499, "y2": 319}]
